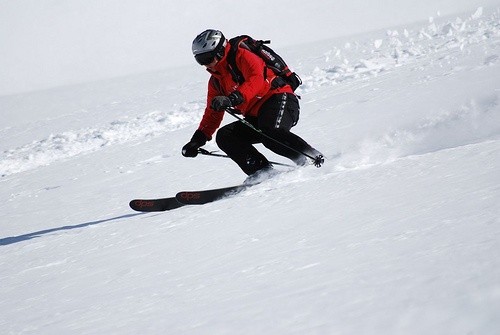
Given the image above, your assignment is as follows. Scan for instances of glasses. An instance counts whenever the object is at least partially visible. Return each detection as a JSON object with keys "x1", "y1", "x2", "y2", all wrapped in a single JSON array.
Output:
[{"x1": 194, "y1": 52, "x2": 215, "y2": 65}]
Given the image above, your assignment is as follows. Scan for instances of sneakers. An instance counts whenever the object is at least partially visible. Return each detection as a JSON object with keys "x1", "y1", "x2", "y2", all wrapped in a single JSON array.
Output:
[{"x1": 243, "y1": 166, "x2": 276, "y2": 184}]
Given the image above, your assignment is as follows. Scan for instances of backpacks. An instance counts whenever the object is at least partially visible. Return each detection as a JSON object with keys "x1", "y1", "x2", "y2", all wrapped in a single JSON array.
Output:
[{"x1": 210, "y1": 34, "x2": 302, "y2": 95}]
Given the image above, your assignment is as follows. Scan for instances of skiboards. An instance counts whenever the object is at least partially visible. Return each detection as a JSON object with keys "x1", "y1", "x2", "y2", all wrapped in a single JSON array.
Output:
[{"x1": 129, "y1": 185, "x2": 245, "y2": 211}]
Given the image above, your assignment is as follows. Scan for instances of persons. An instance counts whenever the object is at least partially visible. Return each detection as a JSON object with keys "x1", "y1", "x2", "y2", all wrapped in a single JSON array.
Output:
[{"x1": 181, "y1": 29, "x2": 325, "y2": 187}]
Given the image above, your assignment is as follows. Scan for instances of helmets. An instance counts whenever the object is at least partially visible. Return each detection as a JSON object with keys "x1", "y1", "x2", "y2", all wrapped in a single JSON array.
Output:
[{"x1": 192, "y1": 29, "x2": 227, "y2": 59}]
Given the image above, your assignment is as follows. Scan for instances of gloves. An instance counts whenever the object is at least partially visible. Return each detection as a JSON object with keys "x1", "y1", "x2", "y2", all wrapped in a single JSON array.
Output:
[
  {"x1": 209, "y1": 90, "x2": 244, "y2": 112},
  {"x1": 181, "y1": 130, "x2": 206, "y2": 157}
]
